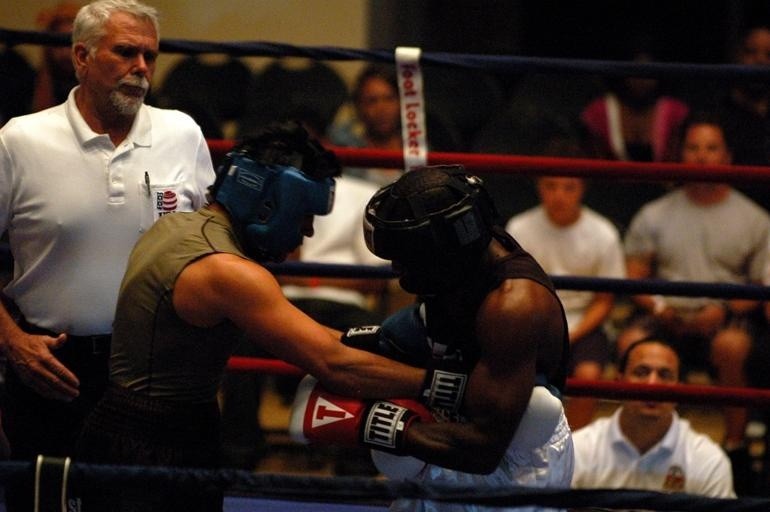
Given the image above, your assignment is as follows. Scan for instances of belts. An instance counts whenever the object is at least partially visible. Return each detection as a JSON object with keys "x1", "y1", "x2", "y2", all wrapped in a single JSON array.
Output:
[{"x1": 20, "y1": 317, "x2": 113, "y2": 361}]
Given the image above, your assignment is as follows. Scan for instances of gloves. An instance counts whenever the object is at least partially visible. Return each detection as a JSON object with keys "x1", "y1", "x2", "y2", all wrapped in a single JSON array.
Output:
[
  {"x1": 339, "y1": 302, "x2": 434, "y2": 370},
  {"x1": 288, "y1": 371, "x2": 437, "y2": 458}
]
